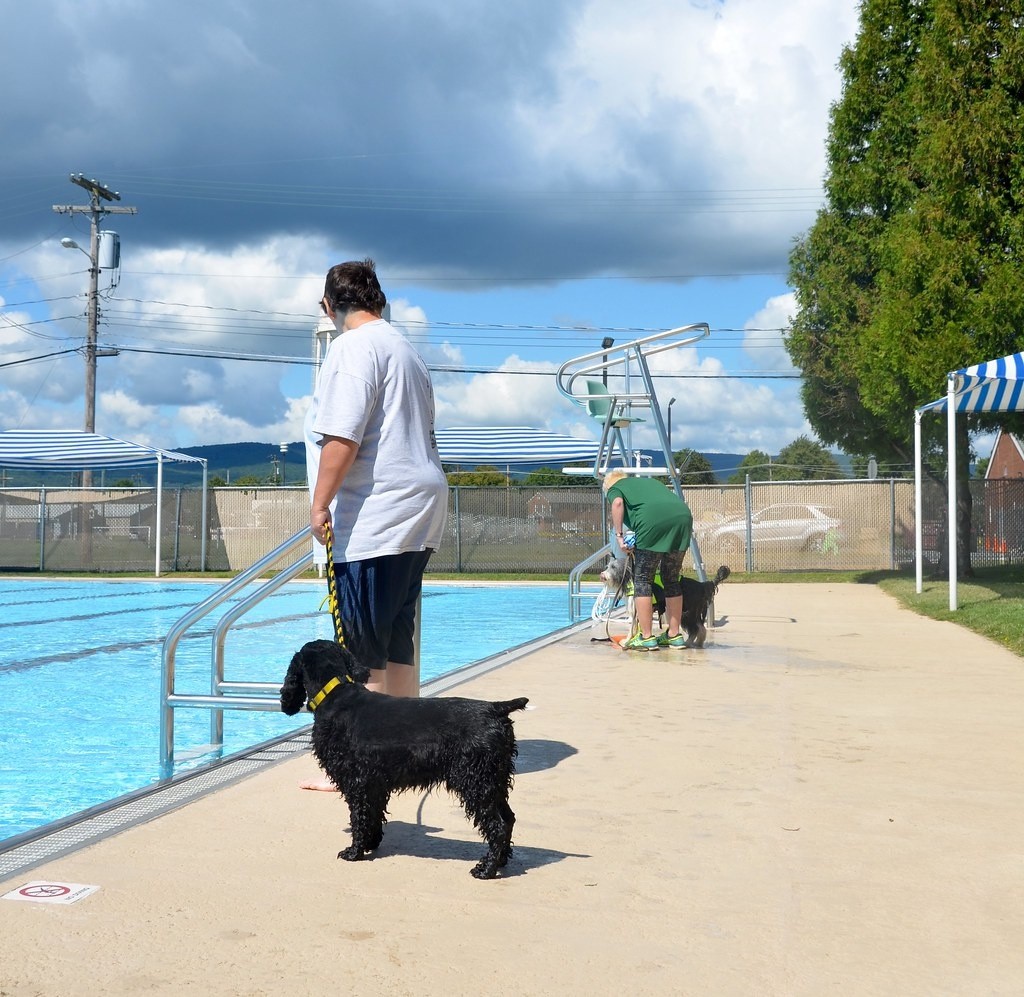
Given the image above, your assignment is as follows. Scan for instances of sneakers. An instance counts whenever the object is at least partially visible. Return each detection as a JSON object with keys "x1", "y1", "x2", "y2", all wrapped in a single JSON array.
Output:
[
  {"x1": 656, "y1": 628, "x2": 687, "y2": 650},
  {"x1": 625, "y1": 633, "x2": 659, "y2": 651}
]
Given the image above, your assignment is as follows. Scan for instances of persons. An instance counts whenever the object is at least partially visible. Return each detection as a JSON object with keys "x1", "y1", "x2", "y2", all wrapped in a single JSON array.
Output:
[
  {"x1": 602, "y1": 470, "x2": 694, "y2": 651},
  {"x1": 298, "y1": 259, "x2": 451, "y2": 793}
]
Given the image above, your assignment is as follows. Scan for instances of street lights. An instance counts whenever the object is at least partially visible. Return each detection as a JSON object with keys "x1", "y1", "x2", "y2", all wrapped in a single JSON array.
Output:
[
  {"x1": 62, "y1": 237, "x2": 97, "y2": 434},
  {"x1": 602, "y1": 336, "x2": 615, "y2": 387}
]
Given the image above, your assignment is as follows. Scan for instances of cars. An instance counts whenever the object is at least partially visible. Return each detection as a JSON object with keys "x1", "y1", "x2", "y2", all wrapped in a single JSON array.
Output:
[
  {"x1": 692, "y1": 505, "x2": 861, "y2": 557},
  {"x1": 700, "y1": 503, "x2": 849, "y2": 555}
]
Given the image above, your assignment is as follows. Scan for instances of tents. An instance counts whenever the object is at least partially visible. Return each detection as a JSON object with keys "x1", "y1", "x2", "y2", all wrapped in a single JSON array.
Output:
[
  {"x1": 914, "y1": 351, "x2": 1024, "y2": 611},
  {"x1": 0, "y1": 430, "x2": 208, "y2": 579}
]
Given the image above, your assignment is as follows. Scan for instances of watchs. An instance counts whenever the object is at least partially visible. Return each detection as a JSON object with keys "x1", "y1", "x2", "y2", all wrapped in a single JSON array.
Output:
[{"x1": 615, "y1": 533, "x2": 623, "y2": 537}]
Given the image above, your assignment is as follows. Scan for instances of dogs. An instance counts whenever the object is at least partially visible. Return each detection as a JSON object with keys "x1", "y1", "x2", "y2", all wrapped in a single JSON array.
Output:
[
  {"x1": 278, "y1": 639, "x2": 529, "y2": 880},
  {"x1": 600, "y1": 556, "x2": 731, "y2": 649}
]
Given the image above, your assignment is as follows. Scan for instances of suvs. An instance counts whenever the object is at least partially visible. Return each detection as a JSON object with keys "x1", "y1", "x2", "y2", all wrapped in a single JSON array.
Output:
[{"x1": 129, "y1": 531, "x2": 141, "y2": 541}]
